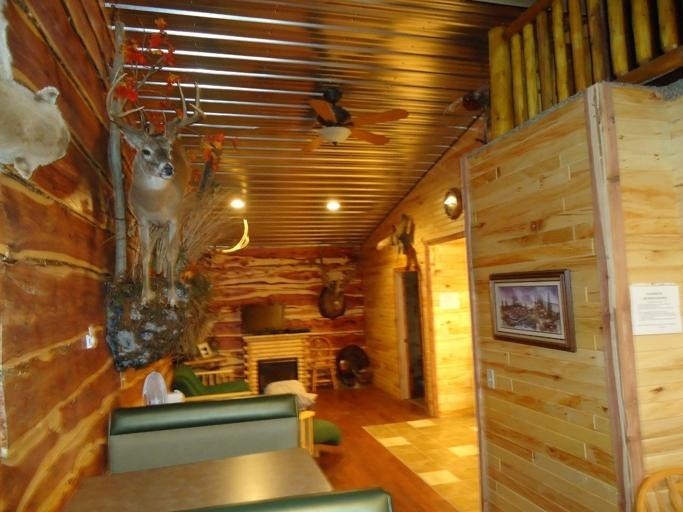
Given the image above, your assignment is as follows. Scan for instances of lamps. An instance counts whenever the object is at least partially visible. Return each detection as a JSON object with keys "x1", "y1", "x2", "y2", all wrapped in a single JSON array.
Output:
[
  {"x1": 318, "y1": 126, "x2": 352, "y2": 147},
  {"x1": 442, "y1": 190, "x2": 462, "y2": 220}
]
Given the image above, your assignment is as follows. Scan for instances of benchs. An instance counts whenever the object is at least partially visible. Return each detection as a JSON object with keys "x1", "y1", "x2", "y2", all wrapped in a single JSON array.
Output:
[
  {"x1": 166, "y1": 486, "x2": 393, "y2": 512},
  {"x1": 106, "y1": 392, "x2": 297, "y2": 471}
]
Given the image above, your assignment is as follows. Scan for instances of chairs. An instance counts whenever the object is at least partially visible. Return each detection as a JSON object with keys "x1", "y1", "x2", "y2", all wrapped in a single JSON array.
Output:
[
  {"x1": 634, "y1": 465, "x2": 683, "y2": 512},
  {"x1": 308, "y1": 336, "x2": 339, "y2": 390}
]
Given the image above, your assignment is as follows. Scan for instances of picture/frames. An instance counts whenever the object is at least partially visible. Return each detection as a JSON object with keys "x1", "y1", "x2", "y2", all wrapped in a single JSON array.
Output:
[
  {"x1": 197, "y1": 342, "x2": 213, "y2": 359},
  {"x1": 487, "y1": 270, "x2": 577, "y2": 353}
]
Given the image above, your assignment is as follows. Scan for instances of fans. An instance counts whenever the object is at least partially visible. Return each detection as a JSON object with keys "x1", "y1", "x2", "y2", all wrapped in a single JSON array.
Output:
[{"x1": 302, "y1": 99, "x2": 408, "y2": 155}]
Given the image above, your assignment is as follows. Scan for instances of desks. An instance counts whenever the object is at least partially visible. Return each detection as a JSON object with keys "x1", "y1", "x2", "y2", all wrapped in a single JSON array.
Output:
[{"x1": 52, "y1": 449, "x2": 332, "y2": 512}]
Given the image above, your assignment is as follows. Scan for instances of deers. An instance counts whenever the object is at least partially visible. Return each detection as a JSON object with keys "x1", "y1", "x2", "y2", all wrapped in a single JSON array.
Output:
[{"x1": 102, "y1": 64, "x2": 209, "y2": 308}]
